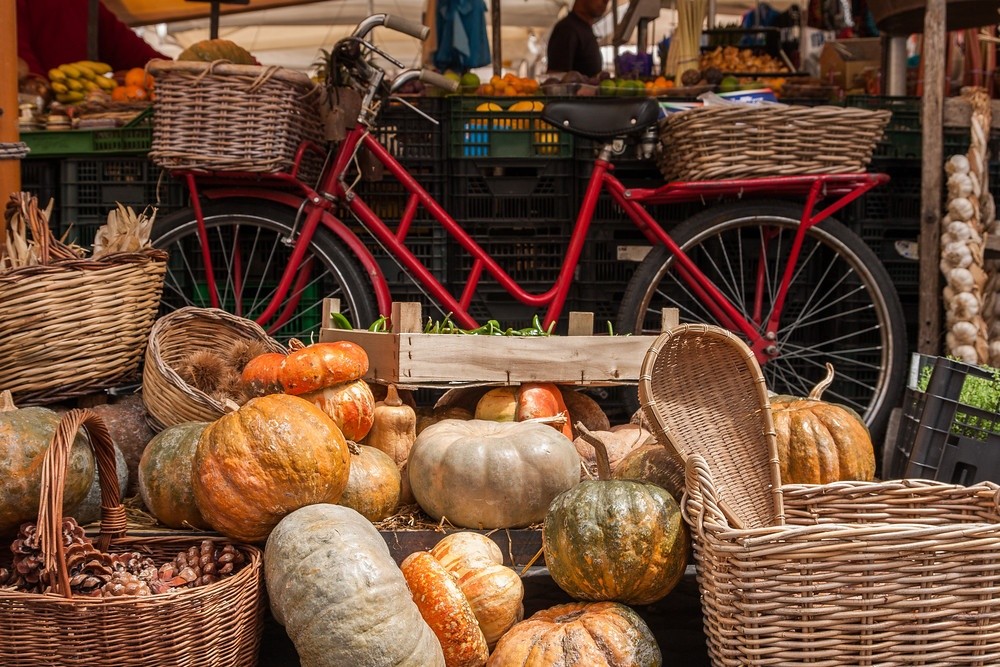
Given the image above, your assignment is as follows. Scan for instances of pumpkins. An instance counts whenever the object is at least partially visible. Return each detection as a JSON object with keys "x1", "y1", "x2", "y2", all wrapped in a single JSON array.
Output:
[
  {"x1": 2, "y1": 332, "x2": 875, "y2": 667},
  {"x1": 176, "y1": 38, "x2": 262, "y2": 65}
]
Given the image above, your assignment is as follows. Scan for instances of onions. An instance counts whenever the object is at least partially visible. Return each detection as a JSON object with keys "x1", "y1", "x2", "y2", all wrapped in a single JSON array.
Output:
[{"x1": 378, "y1": 68, "x2": 443, "y2": 95}]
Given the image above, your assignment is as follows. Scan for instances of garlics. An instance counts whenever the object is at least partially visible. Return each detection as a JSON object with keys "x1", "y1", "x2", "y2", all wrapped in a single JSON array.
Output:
[{"x1": 943, "y1": 152, "x2": 980, "y2": 367}]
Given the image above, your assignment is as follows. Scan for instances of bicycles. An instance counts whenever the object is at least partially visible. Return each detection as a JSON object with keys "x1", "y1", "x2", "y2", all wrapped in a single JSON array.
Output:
[{"x1": 140, "y1": 12, "x2": 906, "y2": 448}]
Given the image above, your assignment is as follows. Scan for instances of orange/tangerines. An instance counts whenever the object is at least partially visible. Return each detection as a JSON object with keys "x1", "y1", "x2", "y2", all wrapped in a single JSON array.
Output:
[
  {"x1": 112, "y1": 66, "x2": 155, "y2": 103},
  {"x1": 476, "y1": 73, "x2": 541, "y2": 96}
]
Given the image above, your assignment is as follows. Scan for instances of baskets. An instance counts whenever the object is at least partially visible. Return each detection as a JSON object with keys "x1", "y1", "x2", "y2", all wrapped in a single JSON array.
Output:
[
  {"x1": 0, "y1": 191, "x2": 169, "y2": 408},
  {"x1": 0, "y1": 408, "x2": 269, "y2": 666},
  {"x1": 637, "y1": 322, "x2": 786, "y2": 528},
  {"x1": 145, "y1": 56, "x2": 330, "y2": 186},
  {"x1": 681, "y1": 452, "x2": 999, "y2": 666},
  {"x1": 650, "y1": 101, "x2": 894, "y2": 181},
  {"x1": 143, "y1": 305, "x2": 290, "y2": 434}
]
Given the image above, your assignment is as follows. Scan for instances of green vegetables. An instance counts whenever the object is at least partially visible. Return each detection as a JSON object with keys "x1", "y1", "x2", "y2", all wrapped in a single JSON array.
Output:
[{"x1": 917, "y1": 353, "x2": 999, "y2": 442}]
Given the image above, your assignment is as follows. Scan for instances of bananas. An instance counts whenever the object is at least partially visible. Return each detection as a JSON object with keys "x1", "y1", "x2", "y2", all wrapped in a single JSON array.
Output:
[{"x1": 48, "y1": 60, "x2": 116, "y2": 107}]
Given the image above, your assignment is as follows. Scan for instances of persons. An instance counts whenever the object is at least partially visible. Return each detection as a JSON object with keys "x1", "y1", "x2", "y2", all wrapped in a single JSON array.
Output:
[{"x1": 546, "y1": 0, "x2": 611, "y2": 78}]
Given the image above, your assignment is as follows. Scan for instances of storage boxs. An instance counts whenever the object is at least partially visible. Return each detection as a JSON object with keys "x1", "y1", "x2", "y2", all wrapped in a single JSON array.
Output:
[
  {"x1": 18, "y1": 86, "x2": 998, "y2": 401},
  {"x1": 890, "y1": 352, "x2": 1000, "y2": 483}
]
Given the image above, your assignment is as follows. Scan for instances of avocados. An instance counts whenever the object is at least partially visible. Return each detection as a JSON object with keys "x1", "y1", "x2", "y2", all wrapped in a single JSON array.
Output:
[
  {"x1": 542, "y1": 71, "x2": 611, "y2": 97},
  {"x1": 682, "y1": 66, "x2": 723, "y2": 97}
]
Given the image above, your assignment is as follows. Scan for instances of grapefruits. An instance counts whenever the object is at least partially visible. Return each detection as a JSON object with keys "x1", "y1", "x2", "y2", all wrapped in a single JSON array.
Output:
[{"x1": 468, "y1": 99, "x2": 557, "y2": 153}]
